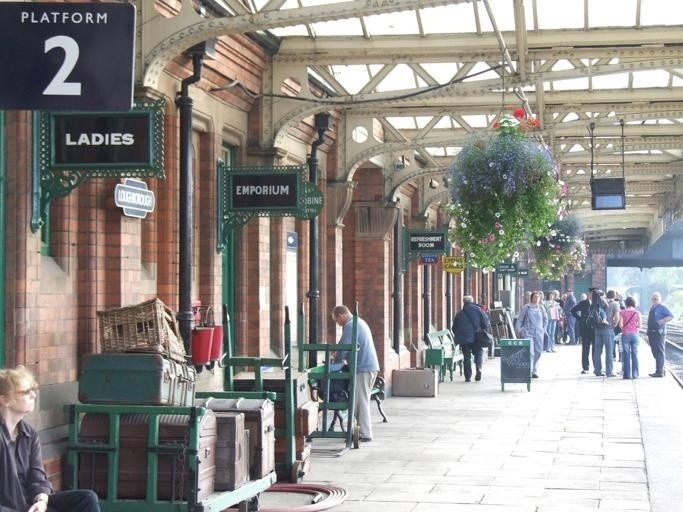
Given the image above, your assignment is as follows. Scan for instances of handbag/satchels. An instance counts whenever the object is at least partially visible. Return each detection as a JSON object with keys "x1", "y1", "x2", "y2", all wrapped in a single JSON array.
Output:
[{"x1": 476, "y1": 329, "x2": 494, "y2": 346}]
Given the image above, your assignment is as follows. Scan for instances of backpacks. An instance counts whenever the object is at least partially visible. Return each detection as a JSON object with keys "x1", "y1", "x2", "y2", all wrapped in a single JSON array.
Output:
[{"x1": 586, "y1": 309, "x2": 602, "y2": 328}]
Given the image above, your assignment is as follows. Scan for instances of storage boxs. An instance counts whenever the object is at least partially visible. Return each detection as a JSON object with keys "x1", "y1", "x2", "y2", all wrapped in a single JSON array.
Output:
[
  {"x1": 213, "y1": 413, "x2": 248, "y2": 490},
  {"x1": 74, "y1": 407, "x2": 216, "y2": 503},
  {"x1": 193, "y1": 396, "x2": 277, "y2": 479}
]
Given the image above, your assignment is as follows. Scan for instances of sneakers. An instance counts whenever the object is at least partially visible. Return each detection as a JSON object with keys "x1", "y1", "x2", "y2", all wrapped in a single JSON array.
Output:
[
  {"x1": 533, "y1": 374, "x2": 538, "y2": 378},
  {"x1": 475, "y1": 370, "x2": 481, "y2": 381},
  {"x1": 648, "y1": 373, "x2": 663, "y2": 377}
]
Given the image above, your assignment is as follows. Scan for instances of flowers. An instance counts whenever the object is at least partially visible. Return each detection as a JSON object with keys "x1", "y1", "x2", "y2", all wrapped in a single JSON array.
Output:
[
  {"x1": 441, "y1": 107, "x2": 568, "y2": 275},
  {"x1": 529, "y1": 215, "x2": 592, "y2": 282}
]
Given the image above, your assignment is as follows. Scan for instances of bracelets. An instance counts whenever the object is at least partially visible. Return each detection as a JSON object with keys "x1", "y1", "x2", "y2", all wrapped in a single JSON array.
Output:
[{"x1": 30, "y1": 497, "x2": 48, "y2": 505}]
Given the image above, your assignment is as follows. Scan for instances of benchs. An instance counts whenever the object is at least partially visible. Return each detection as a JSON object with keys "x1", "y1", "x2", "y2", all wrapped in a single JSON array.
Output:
[
  {"x1": 425, "y1": 329, "x2": 464, "y2": 381},
  {"x1": 309, "y1": 365, "x2": 389, "y2": 431}
]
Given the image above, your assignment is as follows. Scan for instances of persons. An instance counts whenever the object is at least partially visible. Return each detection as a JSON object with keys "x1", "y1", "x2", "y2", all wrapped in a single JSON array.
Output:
[
  {"x1": 451, "y1": 295, "x2": 491, "y2": 382},
  {"x1": 515, "y1": 287, "x2": 642, "y2": 380},
  {"x1": 330, "y1": 305, "x2": 380, "y2": 441},
  {"x1": 645, "y1": 291, "x2": 674, "y2": 377},
  {"x1": 1, "y1": 363, "x2": 99, "y2": 512}
]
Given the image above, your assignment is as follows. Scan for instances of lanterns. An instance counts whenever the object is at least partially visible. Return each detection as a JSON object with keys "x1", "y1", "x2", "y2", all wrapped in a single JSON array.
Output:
[{"x1": 492, "y1": 105, "x2": 542, "y2": 130}]
